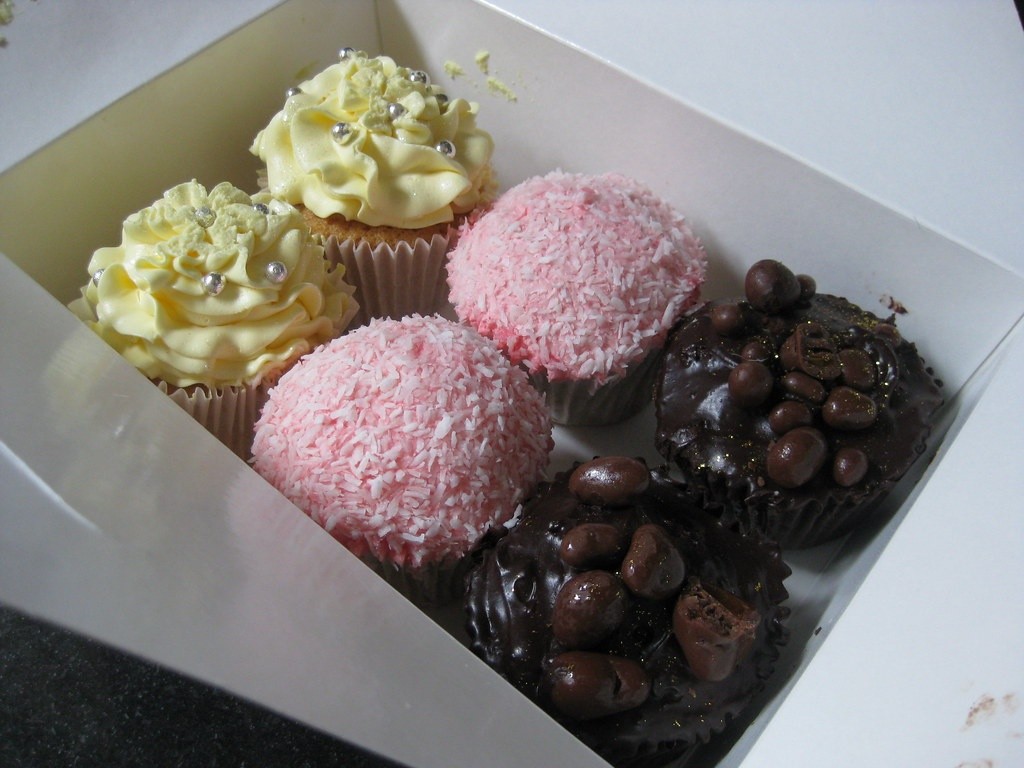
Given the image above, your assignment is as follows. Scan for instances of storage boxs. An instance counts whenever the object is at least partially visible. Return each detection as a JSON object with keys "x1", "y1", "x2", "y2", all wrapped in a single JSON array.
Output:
[{"x1": 1, "y1": 1, "x2": 1024, "y2": 768}]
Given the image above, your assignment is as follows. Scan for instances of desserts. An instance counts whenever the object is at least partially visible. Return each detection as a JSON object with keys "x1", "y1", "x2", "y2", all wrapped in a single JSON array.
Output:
[
  {"x1": 443, "y1": 453, "x2": 793, "y2": 768},
  {"x1": 246, "y1": 46, "x2": 502, "y2": 325},
  {"x1": 447, "y1": 169, "x2": 711, "y2": 429},
  {"x1": 249, "y1": 310, "x2": 554, "y2": 609},
  {"x1": 653, "y1": 259, "x2": 942, "y2": 553},
  {"x1": 63, "y1": 178, "x2": 361, "y2": 465}
]
